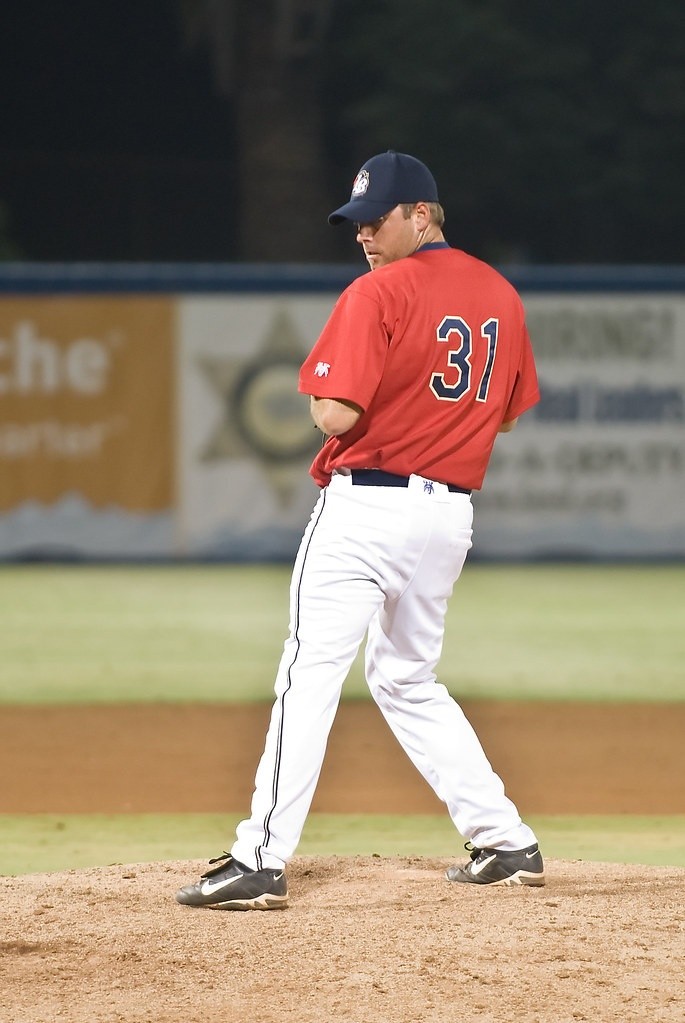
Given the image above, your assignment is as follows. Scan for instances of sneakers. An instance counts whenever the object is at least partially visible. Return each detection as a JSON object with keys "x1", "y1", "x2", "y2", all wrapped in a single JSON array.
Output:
[
  {"x1": 444, "y1": 841, "x2": 546, "y2": 887},
  {"x1": 173, "y1": 850, "x2": 288, "y2": 910}
]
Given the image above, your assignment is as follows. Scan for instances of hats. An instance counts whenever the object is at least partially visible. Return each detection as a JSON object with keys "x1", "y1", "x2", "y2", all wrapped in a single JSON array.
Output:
[{"x1": 328, "y1": 150, "x2": 441, "y2": 224}]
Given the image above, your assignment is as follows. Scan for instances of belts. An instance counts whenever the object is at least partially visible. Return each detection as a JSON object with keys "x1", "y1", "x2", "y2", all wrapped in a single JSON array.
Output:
[{"x1": 348, "y1": 469, "x2": 472, "y2": 497}]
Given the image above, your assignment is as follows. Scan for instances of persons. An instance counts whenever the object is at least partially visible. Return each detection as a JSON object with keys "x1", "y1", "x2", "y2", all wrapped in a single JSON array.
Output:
[{"x1": 174, "y1": 148, "x2": 547, "y2": 910}]
